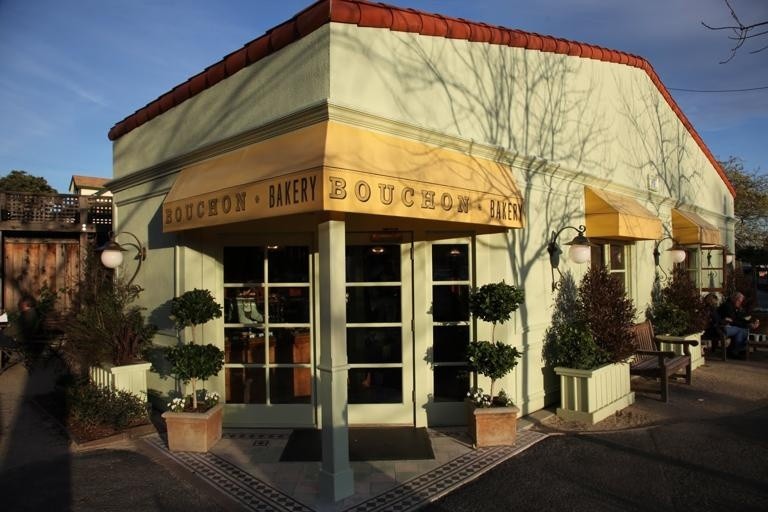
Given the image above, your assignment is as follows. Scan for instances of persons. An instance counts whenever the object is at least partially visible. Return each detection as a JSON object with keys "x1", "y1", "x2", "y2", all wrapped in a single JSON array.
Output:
[
  {"x1": 703, "y1": 292, "x2": 760, "y2": 360},
  {"x1": 16, "y1": 295, "x2": 46, "y2": 352}
]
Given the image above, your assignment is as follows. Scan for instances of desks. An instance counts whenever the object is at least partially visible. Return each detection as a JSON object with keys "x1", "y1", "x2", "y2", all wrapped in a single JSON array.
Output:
[{"x1": 745, "y1": 340, "x2": 768, "y2": 361}]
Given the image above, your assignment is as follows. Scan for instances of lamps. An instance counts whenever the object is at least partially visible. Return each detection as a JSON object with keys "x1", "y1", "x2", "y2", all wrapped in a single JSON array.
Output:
[
  {"x1": 707, "y1": 244, "x2": 736, "y2": 266},
  {"x1": 93, "y1": 231, "x2": 146, "y2": 293},
  {"x1": 547, "y1": 224, "x2": 599, "y2": 291},
  {"x1": 653, "y1": 223, "x2": 691, "y2": 265}
]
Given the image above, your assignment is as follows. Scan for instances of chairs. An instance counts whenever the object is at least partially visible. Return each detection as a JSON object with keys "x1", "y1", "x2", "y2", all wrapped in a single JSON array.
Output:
[
  {"x1": 628, "y1": 319, "x2": 699, "y2": 403},
  {"x1": 700, "y1": 327, "x2": 735, "y2": 361}
]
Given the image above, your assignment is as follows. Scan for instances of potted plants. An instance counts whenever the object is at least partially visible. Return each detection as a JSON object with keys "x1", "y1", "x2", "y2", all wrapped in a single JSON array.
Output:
[
  {"x1": 462, "y1": 279, "x2": 525, "y2": 447},
  {"x1": 83, "y1": 278, "x2": 159, "y2": 404},
  {"x1": 143, "y1": 288, "x2": 226, "y2": 454},
  {"x1": 645, "y1": 264, "x2": 705, "y2": 380},
  {"x1": 541, "y1": 260, "x2": 642, "y2": 425}
]
